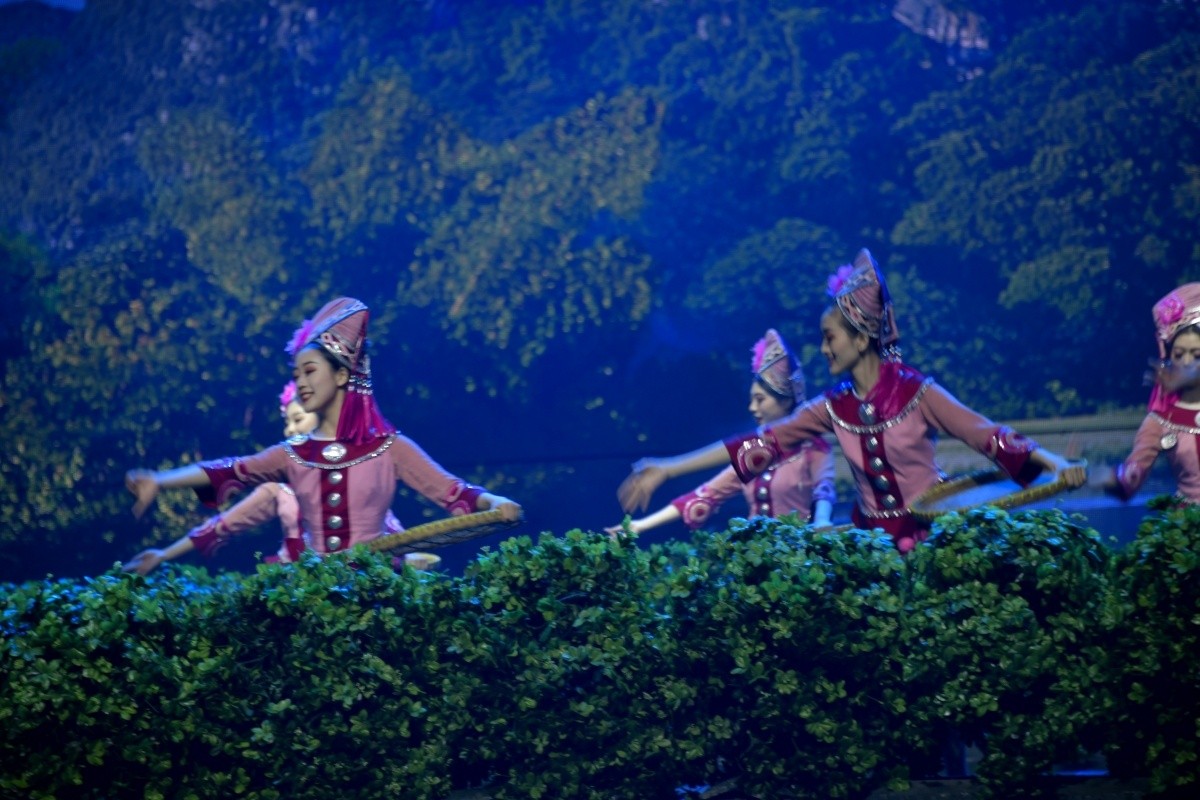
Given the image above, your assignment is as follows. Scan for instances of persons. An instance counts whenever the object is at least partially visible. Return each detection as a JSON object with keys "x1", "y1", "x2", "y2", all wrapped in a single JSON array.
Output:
[
  {"x1": 124, "y1": 293, "x2": 522, "y2": 576},
  {"x1": 1088, "y1": 282, "x2": 1200, "y2": 506},
  {"x1": 604, "y1": 327, "x2": 837, "y2": 537},
  {"x1": 132, "y1": 379, "x2": 405, "y2": 579},
  {"x1": 615, "y1": 247, "x2": 1088, "y2": 555}
]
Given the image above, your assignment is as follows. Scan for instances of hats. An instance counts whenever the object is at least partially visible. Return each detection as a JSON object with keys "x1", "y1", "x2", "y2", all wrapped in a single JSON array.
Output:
[
  {"x1": 1148, "y1": 281, "x2": 1200, "y2": 412},
  {"x1": 751, "y1": 328, "x2": 807, "y2": 403},
  {"x1": 828, "y1": 248, "x2": 920, "y2": 419},
  {"x1": 286, "y1": 297, "x2": 383, "y2": 446}
]
